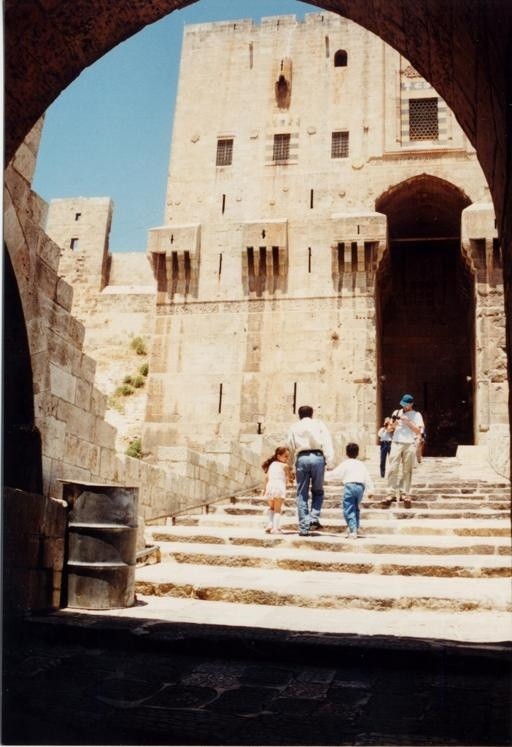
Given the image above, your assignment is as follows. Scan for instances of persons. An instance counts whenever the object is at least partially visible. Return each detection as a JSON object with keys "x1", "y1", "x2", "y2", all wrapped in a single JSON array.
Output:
[
  {"x1": 324, "y1": 441, "x2": 372, "y2": 539},
  {"x1": 382, "y1": 394, "x2": 424, "y2": 506},
  {"x1": 287, "y1": 405, "x2": 333, "y2": 535},
  {"x1": 263, "y1": 447, "x2": 293, "y2": 533},
  {"x1": 414, "y1": 438, "x2": 425, "y2": 468},
  {"x1": 378, "y1": 416, "x2": 402, "y2": 480}
]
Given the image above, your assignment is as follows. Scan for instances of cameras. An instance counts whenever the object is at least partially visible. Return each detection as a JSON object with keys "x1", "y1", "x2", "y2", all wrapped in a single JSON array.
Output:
[{"x1": 392, "y1": 415, "x2": 400, "y2": 420}]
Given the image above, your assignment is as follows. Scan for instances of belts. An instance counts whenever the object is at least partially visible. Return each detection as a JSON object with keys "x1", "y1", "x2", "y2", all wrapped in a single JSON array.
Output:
[{"x1": 298, "y1": 449, "x2": 322, "y2": 453}]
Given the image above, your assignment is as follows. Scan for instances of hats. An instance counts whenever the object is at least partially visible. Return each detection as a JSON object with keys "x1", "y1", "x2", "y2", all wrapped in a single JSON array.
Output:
[{"x1": 399, "y1": 393, "x2": 414, "y2": 408}]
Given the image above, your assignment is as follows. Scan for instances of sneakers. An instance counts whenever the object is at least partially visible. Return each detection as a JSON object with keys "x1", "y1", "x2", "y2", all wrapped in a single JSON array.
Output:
[
  {"x1": 381, "y1": 496, "x2": 396, "y2": 506},
  {"x1": 309, "y1": 520, "x2": 322, "y2": 527},
  {"x1": 298, "y1": 531, "x2": 308, "y2": 536},
  {"x1": 265, "y1": 527, "x2": 282, "y2": 534},
  {"x1": 345, "y1": 525, "x2": 358, "y2": 539},
  {"x1": 399, "y1": 494, "x2": 411, "y2": 508}
]
